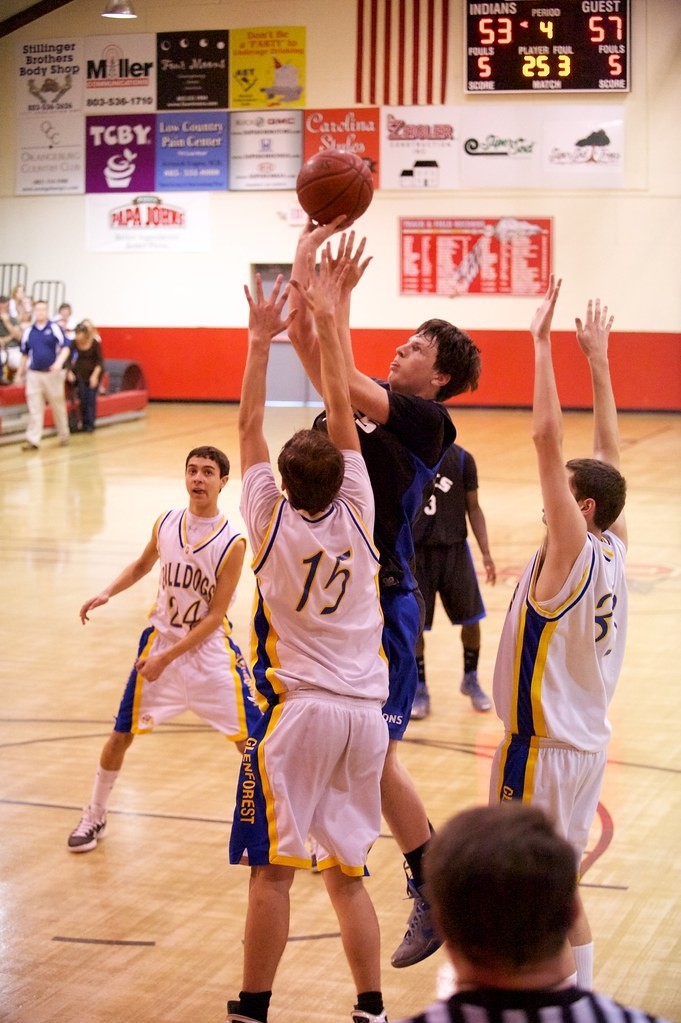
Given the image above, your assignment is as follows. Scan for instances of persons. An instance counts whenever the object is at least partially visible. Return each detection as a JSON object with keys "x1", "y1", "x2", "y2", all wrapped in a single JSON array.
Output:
[
  {"x1": 397, "y1": 803, "x2": 666, "y2": 1023},
  {"x1": 225, "y1": 248, "x2": 394, "y2": 1023},
  {"x1": 490, "y1": 275, "x2": 633, "y2": 993},
  {"x1": 0, "y1": 286, "x2": 106, "y2": 451},
  {"x1": 411, "y1": 445, "x2": 497, "y2": 719},
  {"x1": 287, "y1": 213, "x2": 480, "y2": 968},
  {"x1": 67, "y1": 447, "x2": 264, "y2": 854}
]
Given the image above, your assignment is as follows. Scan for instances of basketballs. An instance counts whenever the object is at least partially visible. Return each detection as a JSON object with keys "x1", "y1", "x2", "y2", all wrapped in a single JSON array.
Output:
[{"x1": 296, "y1": 148, "x2": 374, "y2": 226}]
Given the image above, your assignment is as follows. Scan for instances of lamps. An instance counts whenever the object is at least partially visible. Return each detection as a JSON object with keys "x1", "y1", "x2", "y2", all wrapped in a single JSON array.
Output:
[{"x1": 100, "y1": 0, "x2": 138, "y2": 19}]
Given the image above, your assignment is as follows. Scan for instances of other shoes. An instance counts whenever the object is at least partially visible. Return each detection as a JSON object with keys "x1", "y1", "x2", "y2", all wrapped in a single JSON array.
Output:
[
  {"x1": 350, "y1": 1005, "x2": 388, "y2": 1023},
  {"x1": 224, "y1": 1001, "x2": 267, "y2": 1023}
]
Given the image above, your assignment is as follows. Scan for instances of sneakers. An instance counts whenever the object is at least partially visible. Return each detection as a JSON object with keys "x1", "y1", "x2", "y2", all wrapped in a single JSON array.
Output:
[
  {"x1": 390, "y1": 861, "x2": 444, "y2": 968},
  {"x1": 410, "y1": 683, "x2": 430, "y2": 719},
  {"x1": 67, "y1": 803, "x2": 107, "y2": 852},
  {"x1": 460, "y1": 672, "x2": 492, "y2": 712}
]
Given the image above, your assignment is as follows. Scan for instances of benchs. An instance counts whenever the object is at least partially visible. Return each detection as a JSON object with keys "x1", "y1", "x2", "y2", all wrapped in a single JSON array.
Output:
[{"x1": 0, "y1": 371, "x2": 149, "y2": 444}]
